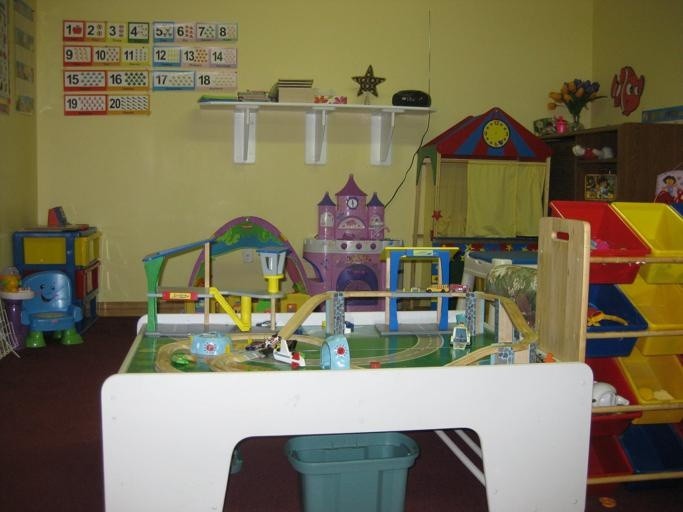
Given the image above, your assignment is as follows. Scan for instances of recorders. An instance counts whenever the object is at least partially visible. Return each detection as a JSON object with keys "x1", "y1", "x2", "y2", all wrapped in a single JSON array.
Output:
[{"x1": 392, "y1": 90, "x2": 431, "y2": 106}]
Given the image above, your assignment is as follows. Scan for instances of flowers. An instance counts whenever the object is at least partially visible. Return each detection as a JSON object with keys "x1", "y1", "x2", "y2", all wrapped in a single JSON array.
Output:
[{"x1": 547, "y1": 78, "x2": 608, "y2": 115}]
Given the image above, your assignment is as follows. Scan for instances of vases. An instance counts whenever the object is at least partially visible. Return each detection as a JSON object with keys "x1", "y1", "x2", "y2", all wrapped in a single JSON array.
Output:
[{"x1": 572, "y1": 113, "x2": 583, "y2": 131}]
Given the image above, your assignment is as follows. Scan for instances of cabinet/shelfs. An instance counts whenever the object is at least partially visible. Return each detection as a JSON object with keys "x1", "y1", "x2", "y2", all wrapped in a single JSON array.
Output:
[{"x1": 539, "y1": 121, "x2": 683, "y2": 218}]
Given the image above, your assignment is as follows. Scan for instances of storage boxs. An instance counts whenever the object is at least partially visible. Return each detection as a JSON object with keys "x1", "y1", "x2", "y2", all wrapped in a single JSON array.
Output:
[{"x1": 24, "y1": 233, "x2": 100, "y2": 298}]
